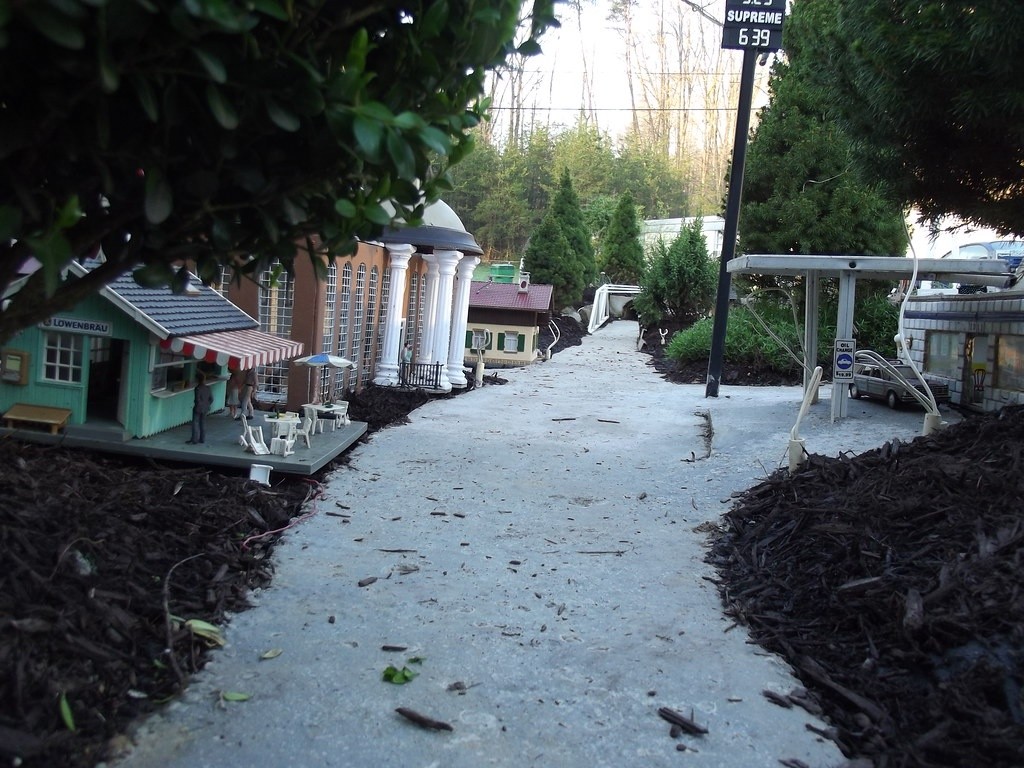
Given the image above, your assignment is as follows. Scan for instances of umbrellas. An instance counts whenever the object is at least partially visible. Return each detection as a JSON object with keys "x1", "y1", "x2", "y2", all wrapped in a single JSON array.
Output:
[{"x1": 293, "y1": 354, "x2": 356, "y2": 404}]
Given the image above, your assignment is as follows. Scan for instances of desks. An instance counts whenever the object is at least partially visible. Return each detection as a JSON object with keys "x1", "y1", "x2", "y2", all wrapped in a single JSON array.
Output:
[
  {"x1": 3, "y1": 404, "x2": 72, "y2": 435},
  {"x1": 264, "y1": 414, "x2": 301, "y2": 440},
  {"x1": 301, "y1": 403, "x2": 345, "y2": 432}
]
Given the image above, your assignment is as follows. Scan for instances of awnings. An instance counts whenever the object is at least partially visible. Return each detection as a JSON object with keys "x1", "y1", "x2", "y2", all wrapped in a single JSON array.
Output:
[{"x1": 160, "y1": 329, "x2": 304, "y2": 371}]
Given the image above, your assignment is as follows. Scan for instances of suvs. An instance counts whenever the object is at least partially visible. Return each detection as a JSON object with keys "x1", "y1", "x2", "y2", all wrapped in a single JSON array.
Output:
[{"x1": 849, "y1": 357, "x2": 952, "y2": 410}]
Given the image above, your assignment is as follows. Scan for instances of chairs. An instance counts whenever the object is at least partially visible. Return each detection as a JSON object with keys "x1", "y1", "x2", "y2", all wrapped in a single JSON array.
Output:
[
  {"x1": 973, "y1": 369, "x2": 986, "y2": 392},
  {"x1": 250, "y1": 464, "x2": 273, "y2": 487},
  {"x1": 238, "y1": 401, "x2": 349, "y2": 457}
]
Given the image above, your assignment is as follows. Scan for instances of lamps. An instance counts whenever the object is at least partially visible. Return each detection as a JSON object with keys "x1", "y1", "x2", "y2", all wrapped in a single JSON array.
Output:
[{"x1": 894, "y1": 333, "x2": 912, "y2": 353}]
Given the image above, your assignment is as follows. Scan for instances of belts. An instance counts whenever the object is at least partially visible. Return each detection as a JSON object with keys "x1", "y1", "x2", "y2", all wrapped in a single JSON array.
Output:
[{"x1": 246, "y1": 384, "x2": 253, "y2": 386}]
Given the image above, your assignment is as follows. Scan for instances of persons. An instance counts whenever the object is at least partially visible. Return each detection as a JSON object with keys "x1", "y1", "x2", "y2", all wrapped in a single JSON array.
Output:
[
  {"x1": 227, "y1": 367, "x2": 240, "y2": 418},
  {"x1": 400, "y1": 343, "x2": 413, "y2": 381},
  {"x1": 187, "y1": 372, "x2": 213, "y2": 442},
  {"x1": 235, "y1": 369, "x2": 257, "y2": 420}
]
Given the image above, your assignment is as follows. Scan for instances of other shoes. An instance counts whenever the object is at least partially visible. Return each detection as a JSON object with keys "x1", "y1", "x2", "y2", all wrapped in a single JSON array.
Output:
[
  {"x1": 235, "y1": 417, "x2": 241, "y2": 421},
  {"x1": 246, "y1": 416, "x2": 253, "y2": 420},
  {"x1": 233, "y1": 415, "x2": 237, "y2": 418},
  {"x1": 228, "y1": 413, "x2": 233, "y2": 416},
  {"x1": 185, "y1": 441, "x2": 197, "y2": 444},
  {"x1": 199, "y1": 441, "x2": 204, "y2": 443}
]
